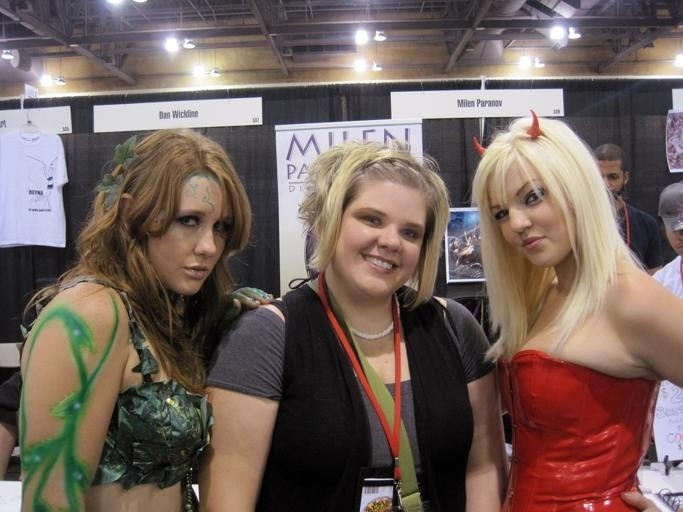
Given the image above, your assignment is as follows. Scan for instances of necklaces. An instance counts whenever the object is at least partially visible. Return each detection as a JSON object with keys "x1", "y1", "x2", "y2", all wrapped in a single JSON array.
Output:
[{"x1": 349, "y1": 292, "x2": 401, "y2": 340}]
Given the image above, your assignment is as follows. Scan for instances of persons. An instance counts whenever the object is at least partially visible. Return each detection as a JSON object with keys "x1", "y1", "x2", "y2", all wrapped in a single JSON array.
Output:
[
  {"x1": 645, "y1": 179, "x2": 682, "y2": 296},
  {"x1": 0, "y1": 369, "x2": 26, "y2": 481},
  {"x1": 471, "y1": 108, "x2": 683, "y2": 512},
  {"x1": 198, "y1": 136, "x2": 509, "y2": 512},
  {"x1": 18, "y1": 123, "x2": 276, "y2": 512},
  {"x1": 591, "y1": 143, "x2": 660, "y2": 278}
]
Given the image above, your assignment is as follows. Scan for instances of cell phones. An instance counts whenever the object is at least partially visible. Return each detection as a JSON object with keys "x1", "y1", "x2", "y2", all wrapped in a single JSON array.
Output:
[{"x1": 355, "y1": 478, "x2": 395, "y2": 512}]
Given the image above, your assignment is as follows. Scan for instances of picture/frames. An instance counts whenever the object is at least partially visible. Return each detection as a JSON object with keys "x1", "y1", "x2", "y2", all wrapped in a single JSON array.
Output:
[{"x1": 441, "y1": 205, "x2": 486, "y2": 287}]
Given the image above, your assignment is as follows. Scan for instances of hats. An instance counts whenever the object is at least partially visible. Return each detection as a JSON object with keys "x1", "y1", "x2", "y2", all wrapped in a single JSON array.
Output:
[{"x1": 657, "y1": 181, "x2": 683, "y2": 231}]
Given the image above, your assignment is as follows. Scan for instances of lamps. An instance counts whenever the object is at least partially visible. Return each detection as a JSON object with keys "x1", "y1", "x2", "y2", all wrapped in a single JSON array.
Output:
[
  {"x1": 183, "y1": 40, "x2": 196, "y2": 50},
  {"x1": 567, "y1": 27, "x2": 582, "y2": 39},
  {"x1": 371, "y1": 63, "x2": 384, "y2": 73},
  {"x1": 374, "y1": 30, "x2": 387, "y2": 42},
  {"x1": 2, "y1": 43, "x2": 17, "y2": 61},
  {"x1": 533, "y1": 56, "x2": 546, "y2": 68},
  {"x1": 54, "y1": 46, "x2": 69, "y2": 87},
  {"x1": 210, "y1": 34, "x2": 220, "y2": 77}
]
